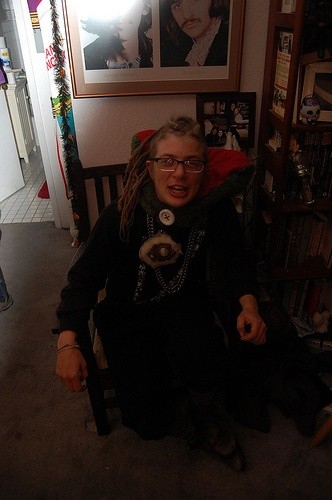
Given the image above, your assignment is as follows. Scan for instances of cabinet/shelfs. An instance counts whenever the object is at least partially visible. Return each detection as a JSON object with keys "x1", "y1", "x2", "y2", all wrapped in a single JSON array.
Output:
[
  {"x1": 4, "y1": 84, "x2": 37, "y2": 164},
  {"x1": 255, "y1": 0, "x2": 332, "y2": 372}
]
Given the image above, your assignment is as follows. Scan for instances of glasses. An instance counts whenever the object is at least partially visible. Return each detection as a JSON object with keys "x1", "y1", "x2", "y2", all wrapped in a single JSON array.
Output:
[{"x1": 147, "y1": 156, "x2": 208, "y2": 174}]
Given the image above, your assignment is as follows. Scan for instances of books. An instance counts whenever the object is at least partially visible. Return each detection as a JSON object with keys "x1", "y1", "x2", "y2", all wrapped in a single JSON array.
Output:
[
  {"x1": 276, "y1": 213, "x2": 332, "y2": 269},
  {"x1": 286, "y1": 130, "x2": 332, "y2": 203},
  {"x1": 280, "y1": 280, "x2": 332, "y2": 318}
]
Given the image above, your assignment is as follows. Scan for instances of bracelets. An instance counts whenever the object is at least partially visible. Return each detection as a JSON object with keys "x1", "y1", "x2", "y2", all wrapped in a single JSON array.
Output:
[{"x1": 56, "y1": 343, "x2": 80, "y2": 352}]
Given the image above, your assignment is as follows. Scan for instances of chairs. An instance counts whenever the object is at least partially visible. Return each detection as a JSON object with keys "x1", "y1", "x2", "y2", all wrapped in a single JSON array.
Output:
[{"x1": 71, "y1": 158, "x2": 251, "y2": 436}]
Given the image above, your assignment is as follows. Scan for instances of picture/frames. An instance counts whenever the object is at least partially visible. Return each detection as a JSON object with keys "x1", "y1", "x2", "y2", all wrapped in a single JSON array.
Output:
[
  {"x1": 196, "y1": 91, "x2": 256, "y2": 150},
  {"x1": 67, "y1": 0, "x2": 247, "y2": 98},
  {"x1": 301, "y1": 62, "x2": 332, "y2": 123}
]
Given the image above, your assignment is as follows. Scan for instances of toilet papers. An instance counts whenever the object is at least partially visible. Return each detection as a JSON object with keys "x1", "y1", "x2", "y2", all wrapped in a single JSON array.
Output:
[{"x1": 6, "y1": 71, "x2": 15, "y2": 84}]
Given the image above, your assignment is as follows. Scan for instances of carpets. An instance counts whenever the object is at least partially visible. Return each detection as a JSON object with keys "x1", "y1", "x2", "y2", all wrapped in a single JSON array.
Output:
[{"x1": 38, "y1": 180, "x2": 50, "y2": 199}]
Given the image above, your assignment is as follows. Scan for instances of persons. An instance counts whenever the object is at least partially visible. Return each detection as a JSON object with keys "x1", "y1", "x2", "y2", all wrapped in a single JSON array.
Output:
[
  {"x1": 205, "y1": 102, "x2": 225, "y2": 114},
  {"x1": 163, "y1": 0, "x2": 228, "y2": 67},
  {"x1": 205, "y1": 126, "x2": 227, "y2": 148},
  {"x1": 52, "y1": 115, "x2": 268, "y2": 463},
  {"x1": 79, "y1": 0, "x2": 153, "y2": 70},
  {"x1": 228, "y1": 103, "x2": 249, "y2": 125}
]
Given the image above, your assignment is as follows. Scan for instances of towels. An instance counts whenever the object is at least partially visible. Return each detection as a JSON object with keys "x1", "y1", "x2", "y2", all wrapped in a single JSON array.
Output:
[{"x1": 0, "y1": 68, "x2": 6, "y2": 85}]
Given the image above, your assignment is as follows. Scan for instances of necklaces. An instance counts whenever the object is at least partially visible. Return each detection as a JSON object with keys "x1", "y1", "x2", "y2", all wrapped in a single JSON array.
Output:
[{"x1": 145, "y1": 212, "x2": 199, "y2": 295}]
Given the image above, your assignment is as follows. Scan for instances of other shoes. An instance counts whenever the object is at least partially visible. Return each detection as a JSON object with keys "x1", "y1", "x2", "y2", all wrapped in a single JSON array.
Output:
[{"x1": 193, "y1": 429, "x2": 246, "y2": 472}]
